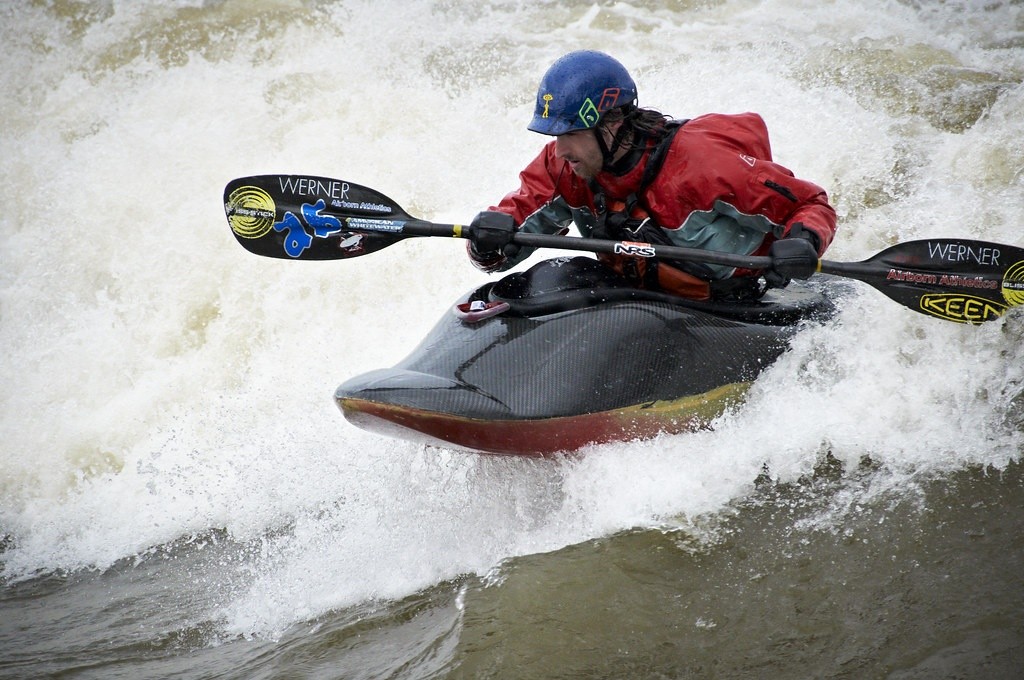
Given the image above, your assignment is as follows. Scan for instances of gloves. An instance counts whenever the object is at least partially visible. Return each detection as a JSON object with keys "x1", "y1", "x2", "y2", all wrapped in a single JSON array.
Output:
[
  {"x1": 469, "y1": 212, "x2": 519, "y2": 247},
  {"x1": 764, "y1": 238, "x2": 819, "y2": 289}
]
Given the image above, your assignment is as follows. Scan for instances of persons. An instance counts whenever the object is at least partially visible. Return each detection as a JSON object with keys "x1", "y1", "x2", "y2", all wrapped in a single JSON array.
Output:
[{"x1": 466, "y1": 50, "x2": 837, "y2": 301}]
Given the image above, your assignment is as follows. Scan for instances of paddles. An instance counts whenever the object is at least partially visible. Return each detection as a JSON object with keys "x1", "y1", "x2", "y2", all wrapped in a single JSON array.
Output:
[{"x1": 221, "y1": 173, "x2": 1023, "y2": 329}]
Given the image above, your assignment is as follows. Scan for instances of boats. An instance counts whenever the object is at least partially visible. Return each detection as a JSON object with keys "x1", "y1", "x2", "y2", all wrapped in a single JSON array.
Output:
[{"x1": 327, "y1": 257, "x2": 864, "y2": 457}]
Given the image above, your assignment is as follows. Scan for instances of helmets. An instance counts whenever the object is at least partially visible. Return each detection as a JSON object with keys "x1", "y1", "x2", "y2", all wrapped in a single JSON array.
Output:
[{"x1": 526, "y1": 49, "x2": 637, "y2": 135}]
